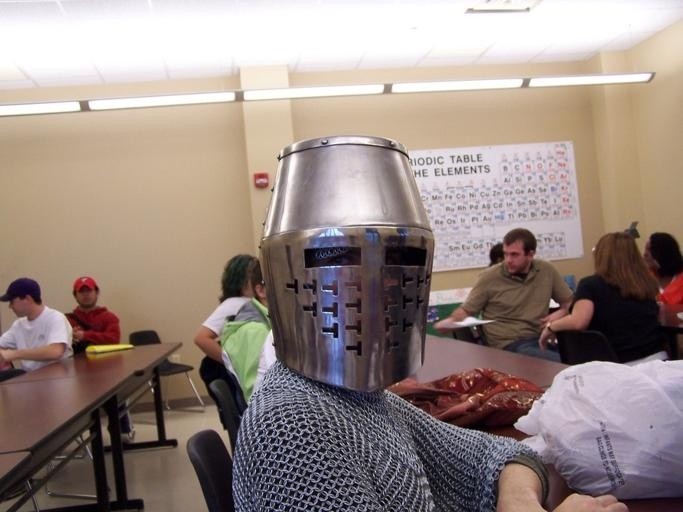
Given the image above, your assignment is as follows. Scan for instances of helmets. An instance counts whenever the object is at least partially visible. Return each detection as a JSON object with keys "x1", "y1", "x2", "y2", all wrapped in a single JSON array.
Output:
[{"x1": 260, "y1": 133, "x2": 436, "y2": 394}]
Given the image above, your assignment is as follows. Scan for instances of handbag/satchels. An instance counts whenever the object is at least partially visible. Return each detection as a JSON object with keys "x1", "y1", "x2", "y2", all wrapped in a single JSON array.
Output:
[{"x1": 388, "y1": 366, "x2": 544, "y2": 430}]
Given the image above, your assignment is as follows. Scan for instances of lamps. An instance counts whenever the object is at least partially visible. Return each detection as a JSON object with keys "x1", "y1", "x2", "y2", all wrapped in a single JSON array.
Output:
[{"x1": 0, "y1": 69, "x2": 658, "y2": 122}]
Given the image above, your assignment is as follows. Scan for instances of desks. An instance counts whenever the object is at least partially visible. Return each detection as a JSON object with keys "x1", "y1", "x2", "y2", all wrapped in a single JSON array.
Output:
[
  {"x1": 399, "y1": 333, "x2": 681, "y2": 511},
  {"x1": 0, "y1": 340, "x2": 193, "y2": 509}
]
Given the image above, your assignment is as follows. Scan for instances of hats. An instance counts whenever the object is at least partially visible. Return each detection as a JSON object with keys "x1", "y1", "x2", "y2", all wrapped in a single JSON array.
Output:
[
  {"x1": 72, "y1": 276, "x2": 99, "y2": 291},
  {"x1": 0, "y1": 278, "x2": 42, "y2": 302}
]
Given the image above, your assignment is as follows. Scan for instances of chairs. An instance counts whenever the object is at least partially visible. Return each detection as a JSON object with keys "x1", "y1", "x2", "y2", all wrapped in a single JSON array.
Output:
[
  {"x1": 184, "y1": 352, "x2": 252, "y2": 512},
  {"x1": 450, "y1": 320, "x2": 488, "y2": 345},
  {"x1": 552, "y1": 328, "x2": 609, "y2": 367},
  {"x1": 126, "y1": 328, "x2": 206, "y2": 418}
]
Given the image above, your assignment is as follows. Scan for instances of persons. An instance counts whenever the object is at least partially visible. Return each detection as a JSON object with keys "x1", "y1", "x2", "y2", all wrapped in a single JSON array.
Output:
[
  {"x1": 434, "y1": 229, "x2": 682, "y2": 363},
  {"x1": 231, "y1": 248, "x2": 629, "y2": 510},
  {"x1": 1, "y1": 277, "x2": 75, "y2": 381},
  {"x1": 64, "y1": 277, "x2": 133, "y2": 444},
  {"x1": 193, "y1": 255, "x2": 278, "y2": 420}
]
